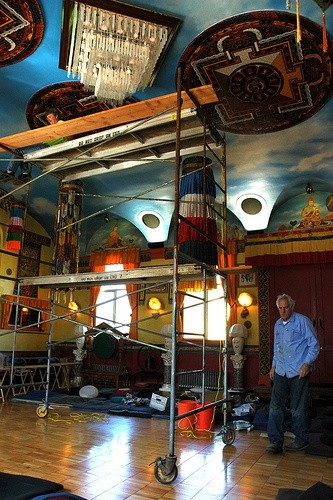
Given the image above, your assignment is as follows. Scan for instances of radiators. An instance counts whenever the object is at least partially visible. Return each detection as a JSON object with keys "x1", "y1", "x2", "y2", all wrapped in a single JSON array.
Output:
[
  {"x1": 177, "y1": 369, "x2": 226, "y2": 390},
  {"x1": 91, "y1": 364, "x2": 128, "y2": 383}
]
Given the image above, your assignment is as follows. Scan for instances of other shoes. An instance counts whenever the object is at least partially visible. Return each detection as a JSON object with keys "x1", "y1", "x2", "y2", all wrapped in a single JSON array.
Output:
[
  {"x1": 265, "y1": 439, "x2": 282, "y2": 453},
  {"x1": 284, "y1": 440, "x2": 308, "y2": 450}
]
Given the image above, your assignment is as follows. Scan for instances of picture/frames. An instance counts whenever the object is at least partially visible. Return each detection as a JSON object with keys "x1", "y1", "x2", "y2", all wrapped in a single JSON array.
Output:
[
  {"x1": 168, "y1": 282, "x2": 172, "y2": 300},
  {"x1": 237, "y1": 272, "x2": 258, "y2": 287},
  {"x1": 138, "y1": 283, "x2": 146, "y2": 301}
]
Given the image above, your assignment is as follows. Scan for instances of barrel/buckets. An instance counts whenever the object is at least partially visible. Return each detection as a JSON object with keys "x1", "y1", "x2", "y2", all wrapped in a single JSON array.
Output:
[
  {"x1": 195, "y1": 402, "x2": 214, "y2": 431},
  {"x1": 177, "y1": 400, "x2": 196, "y2": 431}
]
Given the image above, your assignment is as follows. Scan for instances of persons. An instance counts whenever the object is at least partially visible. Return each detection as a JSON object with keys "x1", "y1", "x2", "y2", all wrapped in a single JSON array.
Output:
[{"x1": 265, "y1": 294, "x2": 320, "y2": 453}]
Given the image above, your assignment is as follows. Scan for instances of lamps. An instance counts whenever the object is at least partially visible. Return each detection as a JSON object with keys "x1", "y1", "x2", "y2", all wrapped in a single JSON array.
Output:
[
  {"x1": 238, "y1": 292, "x2": 255, "y2": 319},
  {"x1": 149, "y1": 298, "x2": 164, "y2": 320},
  {"x1": 67, "y1": 302, "x2": 80, "y2": 321},
  {"x1": 58, "y1": 0, "x2": 183, "y2": 106}
]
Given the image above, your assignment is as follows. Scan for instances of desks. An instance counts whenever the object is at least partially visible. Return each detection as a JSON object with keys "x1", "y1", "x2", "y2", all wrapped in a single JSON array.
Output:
[{"x1": 80, "y1": 370, "x2": 130, "y2": 390}]
